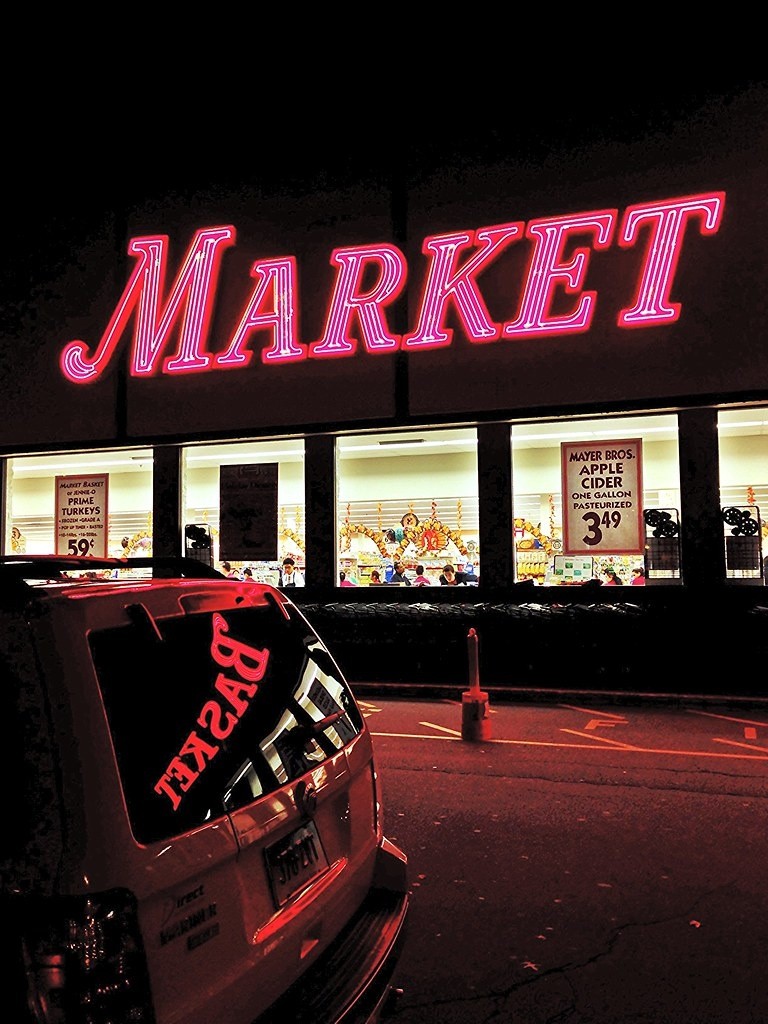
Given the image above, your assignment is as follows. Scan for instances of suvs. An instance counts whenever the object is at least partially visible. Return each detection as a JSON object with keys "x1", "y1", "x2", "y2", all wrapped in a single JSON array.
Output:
[{"x1": 0, "y1": 550, "x2": 416, "y2": 1024}]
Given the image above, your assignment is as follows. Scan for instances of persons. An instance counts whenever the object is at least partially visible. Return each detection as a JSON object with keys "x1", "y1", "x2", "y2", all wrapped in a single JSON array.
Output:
[
  {"x1": 413, "y1": 565, "x2": 430, "y2": 585},
  {"x1": 603, "y1": 568, "x2": 623, "y2": 585},
  {"x1": 371, "y1": 571, "x2": 380, "y2": 583},
  {"x1": 391, "y1": 562, "x2": 411, "y2": 586},
  {"x1": 630, "y1": 567, "x2": 645, "y2": 586},
  {"x1": 439, "y1": 565, "x2": 467, "y2": 585},
  {"x1": 221, "y1": 561, "x2": 236, "y2": 578},
  {"x1": 282, "y1": 558, "x2": 305, "y2": 587},
  {"x1": 340, "y1": 572, "x2": 356, "y2": 587},
  {"x1": 243, "y1": 568, "x2": 255, "y2": 582}
]
